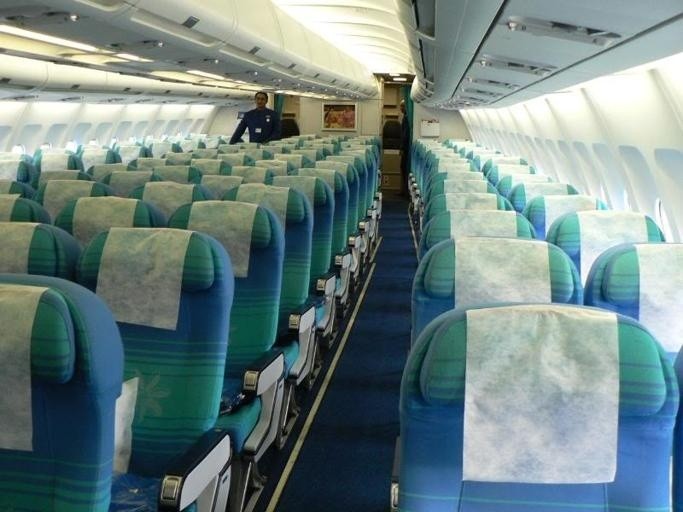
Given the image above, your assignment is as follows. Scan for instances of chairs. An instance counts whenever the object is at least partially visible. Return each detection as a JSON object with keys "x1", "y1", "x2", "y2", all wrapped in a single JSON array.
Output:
[
  {"x1": 389, "y1": 139, "x2": 683, "y2": 512},
  {"x1": 0, "y1": 133, "x2": 381, "y2": 511}
]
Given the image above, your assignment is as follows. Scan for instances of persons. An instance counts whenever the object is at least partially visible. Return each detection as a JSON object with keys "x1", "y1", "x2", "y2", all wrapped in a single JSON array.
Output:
[
  {"x1": 324, "y1": 106, "x2": 339, "y2": 128},
  {"x1": 397, "y1": 99, "x2": 410, "y2": 200},
  {"x1": 337, "y1": 106, "x2": 355, "y2": 129},
  {"x1": 228, "y1": 92, "x2": 282, "y2": 143}
]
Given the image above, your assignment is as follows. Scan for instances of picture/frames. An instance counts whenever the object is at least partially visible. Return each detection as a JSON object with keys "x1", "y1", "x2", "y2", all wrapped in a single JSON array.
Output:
[{"x1": 321, "y1": 101, "x2": 358, "y2": 132}]
